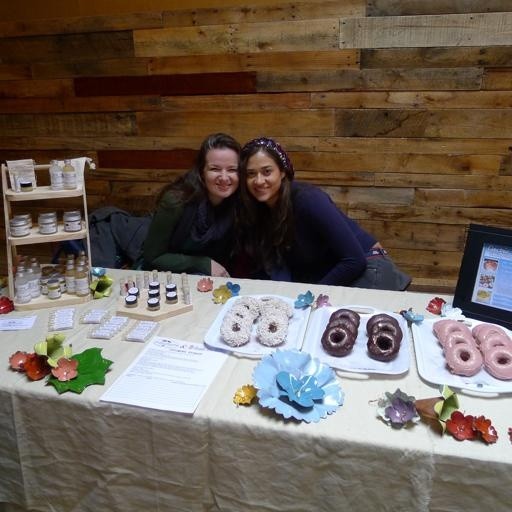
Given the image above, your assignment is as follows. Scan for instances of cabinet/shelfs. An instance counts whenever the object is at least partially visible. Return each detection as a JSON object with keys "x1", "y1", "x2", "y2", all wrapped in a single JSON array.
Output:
[{"x1": 1, "y1": 161, "x2": 94, "y2": 308}]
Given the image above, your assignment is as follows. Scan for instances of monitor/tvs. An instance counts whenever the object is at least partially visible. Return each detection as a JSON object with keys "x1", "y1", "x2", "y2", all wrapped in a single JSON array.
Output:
[{"x1": 451, "y1": 221, "x2": 512, "y2": 330}]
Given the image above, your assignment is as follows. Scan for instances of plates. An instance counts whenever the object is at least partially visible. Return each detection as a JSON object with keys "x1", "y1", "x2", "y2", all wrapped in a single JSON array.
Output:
[
  {"x1": 299, "y1": 305, "x2": 409, "y2": 375},
  {"x1": 202, "y1": 294, "x2": 312, "y2": 360},
  {"x1": 410, "y1": 315, "x2": 512, "y2": 394}
]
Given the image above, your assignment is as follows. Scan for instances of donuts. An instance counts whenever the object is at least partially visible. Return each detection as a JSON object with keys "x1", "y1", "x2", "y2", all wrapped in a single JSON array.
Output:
[
  {"x1": 220, "y1": 295, "x2": 294, "y2": 347},
  {"x1": 432, "y1": 320, "x2": 512, "y2": 380},
  {"x1": 321, "y1": 309, "x2": 360, "y2": 357},
  {"x1": 366, "y1": 314, "x2": 403, "y2": 362}
]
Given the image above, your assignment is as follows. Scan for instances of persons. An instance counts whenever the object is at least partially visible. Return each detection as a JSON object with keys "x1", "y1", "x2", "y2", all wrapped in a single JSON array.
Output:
[
  {"x1": 128, "y1": 132, "x2": 247, "y2": 280},
  {"x1": 232, "y1": 136, "x2": 413, "y2": 293}
]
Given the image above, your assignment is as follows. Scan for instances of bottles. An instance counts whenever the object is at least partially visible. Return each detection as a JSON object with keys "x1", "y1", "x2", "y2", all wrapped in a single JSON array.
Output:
[
  {"x1": 20, "y1": 182, "x2": 33, "y2": 192},
  {"x1": 13, "y1": 250, "x2": 91, "y2": 304},
  {"x1": 49, "y1": 158, "x2": 76, "y2": 192}
]
